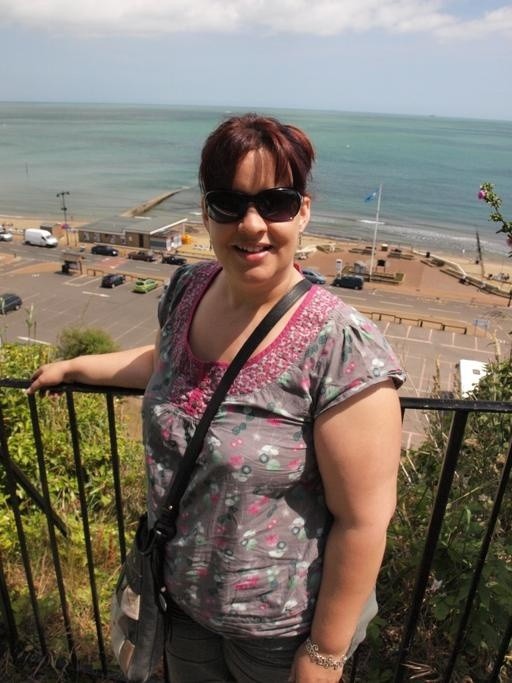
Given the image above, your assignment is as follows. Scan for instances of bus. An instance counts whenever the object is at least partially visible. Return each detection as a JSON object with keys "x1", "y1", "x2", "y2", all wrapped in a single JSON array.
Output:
[{"x1": 455, "y1": 357, "x2": 491, "y2": 404}]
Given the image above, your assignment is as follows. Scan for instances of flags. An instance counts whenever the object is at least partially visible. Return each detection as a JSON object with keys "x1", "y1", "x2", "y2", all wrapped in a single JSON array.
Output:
[{"x1": 364, "y1": 187, "x2": 379, "y2": 202}]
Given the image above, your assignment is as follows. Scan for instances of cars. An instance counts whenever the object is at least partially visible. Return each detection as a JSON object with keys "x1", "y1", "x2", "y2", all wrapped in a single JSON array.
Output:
[
  {"x1": 89, "y1": 243, "x2": 120, "y2": 257},
  {"x1": 0, "y1": 227, "x2": 13, "y2": 242},
  {"x1": 126, "y1": 249, "x2": 159, "y2": 262},
  {"x1": 0, "y1": 292, "x2": 22, "y2": 314},
  {"x1": 134, "y1": 276, "x2": 159, "y2": 294},
  {"x1": 300, "y1": 268, "x2": 327, "y2": 286},
  {"x1": 159, "y1": 252, "x2": 188, "y2": 265},
  {"x1": 99, "y1": 273, "x2": 126, "y2": 289}
]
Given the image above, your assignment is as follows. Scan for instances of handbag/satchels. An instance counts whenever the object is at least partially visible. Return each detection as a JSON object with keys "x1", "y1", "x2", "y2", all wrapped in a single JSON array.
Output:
[{"x1": 108, "y1": 509, "x2": 177, "y2": 683}]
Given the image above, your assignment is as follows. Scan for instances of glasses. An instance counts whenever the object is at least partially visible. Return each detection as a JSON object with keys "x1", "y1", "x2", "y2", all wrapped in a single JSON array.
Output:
[{"x1": 197, "y1": 184, "x2": 305, "y2": 222}]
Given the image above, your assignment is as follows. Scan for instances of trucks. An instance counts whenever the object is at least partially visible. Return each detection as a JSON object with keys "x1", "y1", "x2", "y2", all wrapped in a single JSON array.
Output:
[{"x1": 24, "y1": 228, "x2": 58, "y2": 247}]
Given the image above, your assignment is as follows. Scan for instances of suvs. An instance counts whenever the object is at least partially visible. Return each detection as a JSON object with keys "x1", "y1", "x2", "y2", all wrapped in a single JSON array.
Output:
[{"x1": 332, "y1": 274, "x2": 365, "y2": 292}]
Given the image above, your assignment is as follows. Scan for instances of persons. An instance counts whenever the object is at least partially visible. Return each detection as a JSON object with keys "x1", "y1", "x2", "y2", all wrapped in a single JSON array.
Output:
[{"x1": 24, "y1": 123, "x2": 407, "y2": 682}]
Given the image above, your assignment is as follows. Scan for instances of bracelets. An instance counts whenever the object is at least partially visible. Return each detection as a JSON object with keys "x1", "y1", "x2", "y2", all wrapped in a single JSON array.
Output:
[{"x1": 303, "y1": 636, "x2": 351, "y2": 671}]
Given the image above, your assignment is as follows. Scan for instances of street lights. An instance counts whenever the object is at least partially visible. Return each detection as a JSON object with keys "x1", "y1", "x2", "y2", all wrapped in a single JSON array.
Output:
[{"x1": 56, "y1": 190, "x2": 71, "y2": 245}]
